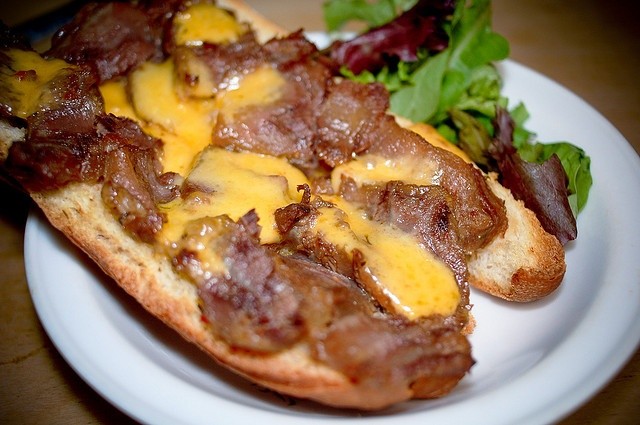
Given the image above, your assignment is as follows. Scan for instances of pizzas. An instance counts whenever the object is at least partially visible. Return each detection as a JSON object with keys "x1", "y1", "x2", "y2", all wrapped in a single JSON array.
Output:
[{"x1": 0, "y1": 0, "x2": 594, "y2": 413}]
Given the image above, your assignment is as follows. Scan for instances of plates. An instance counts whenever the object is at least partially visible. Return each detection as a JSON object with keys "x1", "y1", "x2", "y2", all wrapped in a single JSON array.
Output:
[{"x1": 25, "y1": 31, "x2": 640, "y2": 425}]
